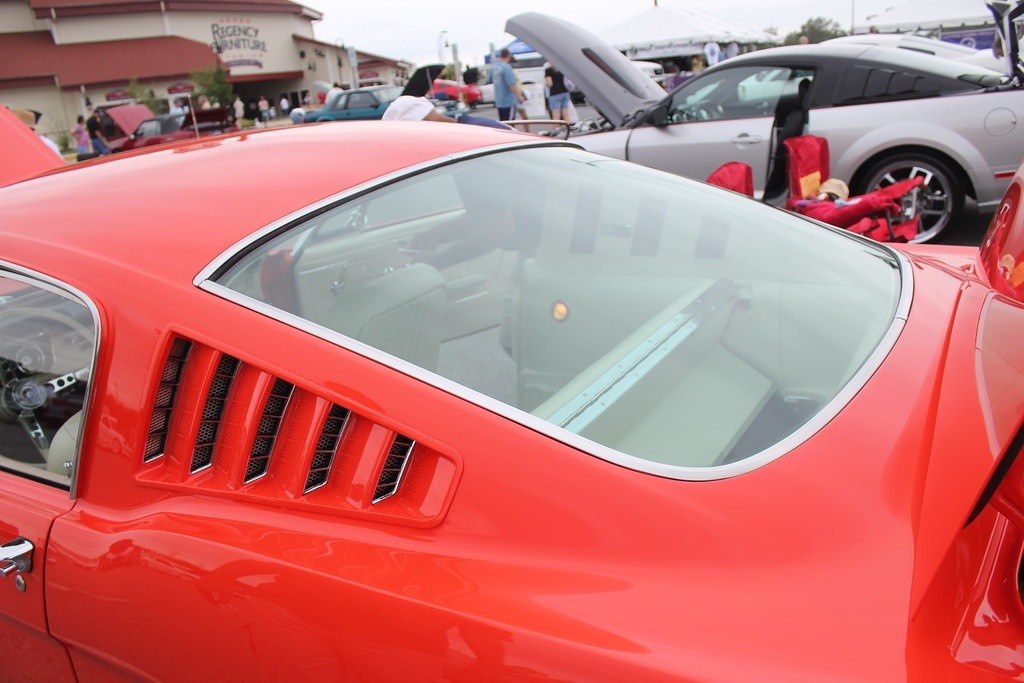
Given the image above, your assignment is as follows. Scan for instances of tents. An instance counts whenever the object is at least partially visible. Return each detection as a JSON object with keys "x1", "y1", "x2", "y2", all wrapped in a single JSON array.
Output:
[
  {"x1": 484, "y1": 38, "x2": 546, "y2": 67},
  {"x1": 599, "y1": 0, "x2": 784, "y2": 50}
]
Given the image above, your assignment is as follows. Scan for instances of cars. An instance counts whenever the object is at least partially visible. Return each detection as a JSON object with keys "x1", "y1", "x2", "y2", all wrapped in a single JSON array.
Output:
[
  {"x1": 105, "y1": 104, "x2": 240, "y2": 154},
  {"x1": 505, "y1": 11, "x2": 1024, "y2": 247},
  {"x1": 305, "y1": 64, "x2": 495, "y2": 123},
  {"x1": 1, "y1": 103, "x2": 1024, "y2": 683}
]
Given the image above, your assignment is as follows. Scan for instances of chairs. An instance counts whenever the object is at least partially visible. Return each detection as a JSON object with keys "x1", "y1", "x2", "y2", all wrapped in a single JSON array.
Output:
[
  {"x1": 45, "y1": 408, "x2": 83, "y2": 477},
  {"x1": 706, "y1": 134, "x2": 924, "y2": 245},
  {"x1": 332, "y1": 261, "x2": 443, "y2": 377},
  {"x1": 798, "y1": 77, "x2": 811, "y2": 108}
]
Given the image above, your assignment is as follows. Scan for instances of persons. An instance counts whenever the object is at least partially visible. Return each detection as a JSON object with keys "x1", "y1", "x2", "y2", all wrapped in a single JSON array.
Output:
[
  {"x1": 303, "y1": 83, "x2": 342, "y2": 105},
  {"x1": 870, "y1": 26, "x2": 881, "y2": 33},
  {"x1": 690, "y1": 55, "x2": 705, "y2": 71},
  {"x1": 983, "y1": 37, "x2": 1005, "y2": 59},
  {"x1": 462, "y1": 66, "x2": 480, "y2": 108},
  {"x1": 86, "y1": 108, "x2": 110, "y2": 154},
  {"x1": 799, "y1": 36, "x2": 809, "y2": 44},
  {"x1": 541, "y1": 60, "x2": 578, "y2": 126},
  {"x1": 198, "y1": 95, "x2": 211, "y2": 110},
  {"x1": 233, "y1": 95, "x2": 291, "y2": 128},
  {"x1": 169, "y1": 98, "x2": 185, "y2": 126},
  {"x1": 71, "y1": 115, "x2": 89, "y2": 154},
  {"x1": 486, "y1": 49, "x2": 530, "y2": 132},
  {"x1": 382, "y1": 94, "x2": 518, "y2": 130}
]
